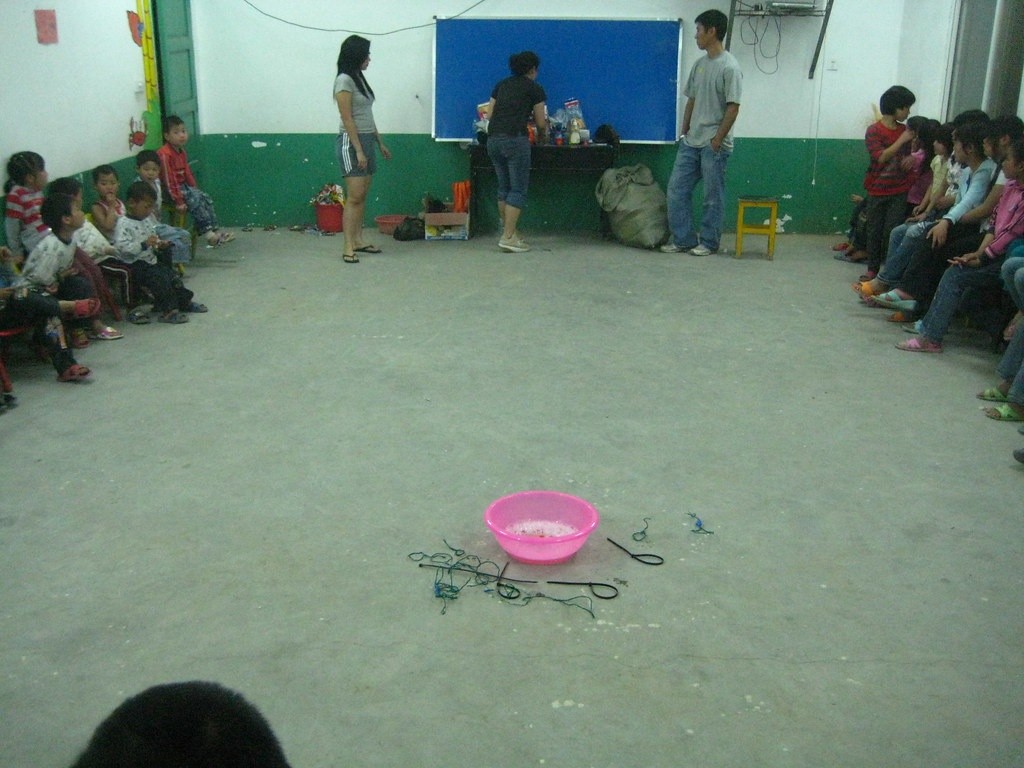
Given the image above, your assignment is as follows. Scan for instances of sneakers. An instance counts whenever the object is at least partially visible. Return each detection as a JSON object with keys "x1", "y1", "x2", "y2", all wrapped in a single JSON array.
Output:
[{"x1": 498, "y1": 231, "x2": 531, "y2": 252}]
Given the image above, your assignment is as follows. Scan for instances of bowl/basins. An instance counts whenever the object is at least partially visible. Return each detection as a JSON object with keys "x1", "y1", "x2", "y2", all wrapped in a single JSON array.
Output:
[
  {"x1": 484, "y1": 491, "x2": 600, "y2": 564},
  {"x1": 375, "y1": 216, "x2": 407, "y2": 234}
]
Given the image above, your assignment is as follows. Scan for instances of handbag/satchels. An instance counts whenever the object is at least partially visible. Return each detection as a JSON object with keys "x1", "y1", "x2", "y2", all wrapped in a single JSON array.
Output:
[{"x1": 393, "y1": 217, "x2": 425, "y2": 241}]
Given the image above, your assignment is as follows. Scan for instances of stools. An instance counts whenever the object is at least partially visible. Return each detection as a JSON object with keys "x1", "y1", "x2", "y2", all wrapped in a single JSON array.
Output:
[{"x1": 736, "y1": 194, "x2": 779, "y2": 261}]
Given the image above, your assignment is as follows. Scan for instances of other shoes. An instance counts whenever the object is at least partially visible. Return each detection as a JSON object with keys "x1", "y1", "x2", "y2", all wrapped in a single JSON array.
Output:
[
  {"x1": 690, "y1": 244, "x2": 712, "y2": 256},
  {"x1": 1002, "y1": 311, "x2": 1022, "y2": 340},
  {"x1": 0, "y1": 394, "x2": 15, "y2": 410},
  {"x1": 660, "y1": 244, "x2": 688, "y2": 253},
  {"x1": 1013, "y1": 447, "x2": 1024, "y2": 463}
]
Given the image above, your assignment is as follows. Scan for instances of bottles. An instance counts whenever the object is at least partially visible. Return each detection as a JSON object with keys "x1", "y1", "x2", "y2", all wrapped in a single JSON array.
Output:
[
  {"x1": 555, "y1": 131, "x2": 563, "y2": 145},
  {"x1": 569, "y1": 118, "x2": 581, "y2": 145}
]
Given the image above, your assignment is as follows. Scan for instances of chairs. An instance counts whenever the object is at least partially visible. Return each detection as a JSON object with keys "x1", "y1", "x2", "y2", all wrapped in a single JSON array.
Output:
[{"x1": 0, "y1": 198, "x2": 200, "y2": 388}]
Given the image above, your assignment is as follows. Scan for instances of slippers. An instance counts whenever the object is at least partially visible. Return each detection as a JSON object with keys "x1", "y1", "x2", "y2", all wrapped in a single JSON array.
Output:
[
  {"x1": 57, "y1": 364, "x2": 91, "y2": 381},
  {"x1": 984, "y1": 404, "x2": 1024, "y2": 422},
  {"x1": 977, "y1": 386, "x2": 1008, "y2": 402},
  {"x1": 62, "y1": 298, "x2": 101, "y2": 320},
  {"x1": 89, "y1": 326, "x2": 123, "y2": 339},
  {"x1": 894, "y1": 336, "x2": 943, "y2": 352},
  {"x1": 343, "y1": 252, "x2": 359, "y2": 263},
  {"x1": 832, "y1": 241, "x2": 921, "y2": 321},
  {"x1": 206, "y1": 234, "x2": 224, "y2": 250},
  {"x1": 353, "y1": 244, "x2": 383, "y2": 253},
  {"x1": 219, "y1": 232, "x2": 235, "y2": 241},
  {"x1": 70, "y1": 332, "x2": 89, "y2": 348},
  {"x1": 127, "y1": 306, "x2": 149, "y2": 323},
  {"x1": 158, "y1": 310, "x2": 189, "y2": 323},
  {"x1": 184, "y1": 302, "x2": 207, "y2": 313}
]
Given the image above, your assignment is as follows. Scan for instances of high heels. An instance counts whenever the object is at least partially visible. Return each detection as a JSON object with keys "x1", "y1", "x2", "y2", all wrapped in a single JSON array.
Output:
[
  {"x1": 871, "y1": 290, "x2": 917, "y2": 311},
  {"x1": 901, "y1": 319, "x2": 923, "y2": 333}
]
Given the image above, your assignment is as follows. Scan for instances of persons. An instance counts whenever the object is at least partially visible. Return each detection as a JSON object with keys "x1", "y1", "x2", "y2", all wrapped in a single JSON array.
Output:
[
  {"x1": 660, "y1": 9, "x2": 744, "y2": 256},
  {"x1": 834, "y1": 85, "x2": 1024, "y2": 468},
  {"x1": 69, "y1": 681, "x2": 291, "y2": 768},
  {"x1": 0, "y1": 115, "x2": 235, "y2": 414},
  {"x1": 487, "y1": 51, "x2": 547, "y2": 253},
  {"x1": 333, "y1": 35, "x2": 391, "y2": 264}
]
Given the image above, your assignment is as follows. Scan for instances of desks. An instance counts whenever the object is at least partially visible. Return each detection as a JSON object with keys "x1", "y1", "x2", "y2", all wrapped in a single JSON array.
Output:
[{"x1": 469, "y1": 145, "x2": 616, "y2": 237}]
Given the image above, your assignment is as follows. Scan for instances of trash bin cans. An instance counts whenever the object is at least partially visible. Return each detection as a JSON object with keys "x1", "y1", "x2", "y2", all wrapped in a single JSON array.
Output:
[{"x1": 315, "y1": 203, "x2": 343, "y2": 233}]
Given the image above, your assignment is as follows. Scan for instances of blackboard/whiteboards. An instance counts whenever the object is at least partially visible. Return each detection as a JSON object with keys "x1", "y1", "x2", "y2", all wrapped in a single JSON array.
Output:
[{"x1": 432, "y1": 14, "x2": 683, "y2": 144}]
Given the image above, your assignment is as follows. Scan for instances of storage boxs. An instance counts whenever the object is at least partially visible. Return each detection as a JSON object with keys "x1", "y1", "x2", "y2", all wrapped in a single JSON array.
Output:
[{"x1": 425, "y1": 202, "x2": 471, "y2": 242}]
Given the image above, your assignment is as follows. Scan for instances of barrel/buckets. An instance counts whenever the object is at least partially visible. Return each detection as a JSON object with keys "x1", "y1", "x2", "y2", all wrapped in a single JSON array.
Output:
[{"x1": 315, "y1": 204, "x2": 343, "y2": 232}]
